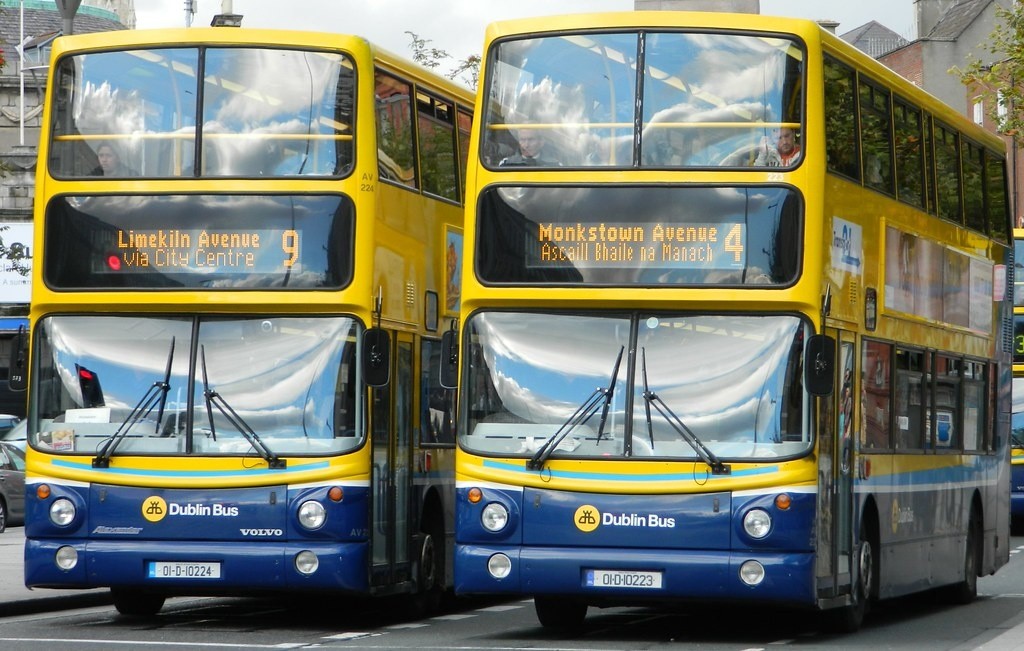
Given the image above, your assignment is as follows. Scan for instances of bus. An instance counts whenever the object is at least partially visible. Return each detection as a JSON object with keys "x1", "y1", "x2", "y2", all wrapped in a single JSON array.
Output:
[
  {"x1": 1011, "y1": 228, "x2": 1024, "y2": 505},
  {"x1": 439, "y1": 13, "x2": 1014, "y2": 629},
  {"x1": 6, "y1": 24, "x2": 478, "y2": 617}
]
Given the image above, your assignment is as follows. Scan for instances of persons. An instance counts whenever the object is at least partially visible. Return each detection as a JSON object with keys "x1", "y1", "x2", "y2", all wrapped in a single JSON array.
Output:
[
  {"x1": 756, "y1": 120, "x2": 800, "y2": 167},
  {"x1": 844, "y1": 384, "x2": 852, "y2": 437},
  {"x1": 86, "y1": 141, "x2": 138, "y2": 176},
  {"x1": 484, "y1": 122, "x2": 564, "y2": 167}
]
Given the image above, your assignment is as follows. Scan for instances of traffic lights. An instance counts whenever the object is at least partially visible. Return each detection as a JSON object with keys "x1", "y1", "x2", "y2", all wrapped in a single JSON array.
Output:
[{"x1": 77, "y1": 363, "x2": 106, "y2": 411}]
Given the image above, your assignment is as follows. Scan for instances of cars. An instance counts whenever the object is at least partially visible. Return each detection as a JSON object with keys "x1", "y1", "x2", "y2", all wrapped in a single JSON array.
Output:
[
  {"x1": 0, "y1": 413, "x2": 21, "y2": 440},
  {"x1": 0, "y1": 417, "x2": 27, "y2": 532}
]
